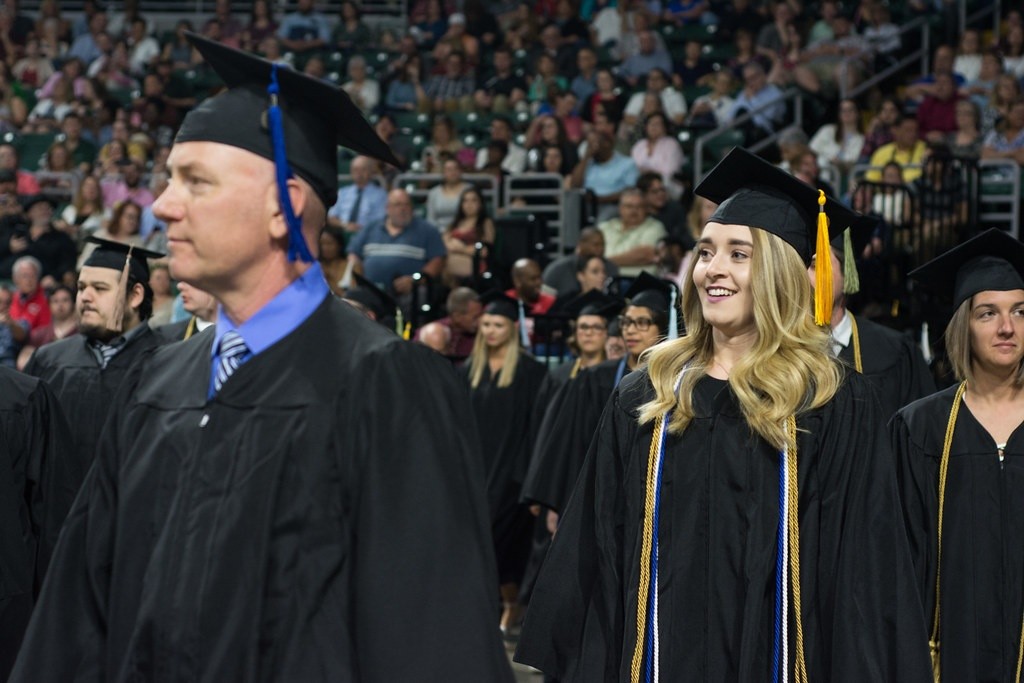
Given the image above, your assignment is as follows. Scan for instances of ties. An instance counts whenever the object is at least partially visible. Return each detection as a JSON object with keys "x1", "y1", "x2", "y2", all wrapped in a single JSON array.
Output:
[
  {"x1": 213, "y1": 329, "x2": 249, "y2": 393},
  {"x1": 88, "y1": 336, "x2": 127, "y2": 370}
]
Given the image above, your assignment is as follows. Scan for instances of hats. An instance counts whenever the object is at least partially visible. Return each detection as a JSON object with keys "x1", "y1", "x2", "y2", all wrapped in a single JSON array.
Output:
[
  {"x1": 627, "y1": 270, "x2": 683, "y2": 340},
  {"x1": 693, "y1": 146, "x2": 863, "y2": 325},
  {"x1": 907, "y1": 228, "x2": 1024, "y2": 321},
  {"x1": 340, "y1": 270, "x2": 403, "y2": 338},
  {"x1": 476, "y1": 288, "x2": 534, "y2": 347},
  {"x1": 173, "y1": 27, "x2": 407, "y2": 263},
  {"x1": 81, "y1": 235, "x2": 167, "y2": 331},
  {"x1": 567, "y1": 287, "x2": 627, "y2": 323},
  {"x1": 829, "y1": 206, "x2": 881, "y2": 293}
]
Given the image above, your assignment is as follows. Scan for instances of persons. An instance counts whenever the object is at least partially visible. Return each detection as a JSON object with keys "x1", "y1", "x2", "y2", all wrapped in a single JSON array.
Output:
[
  {"x1": 0, "y1": 24, "x2": 521, "y2": 683},
  {"x1": 512, "y1": 145, "x2": 933, "y2": 683},
  {"x1": 1, "y1": 0, "x2": 1024, "y2": 681},
  {"x1": 887, "y1": 228, "x2": 1023, "y2": 683}
]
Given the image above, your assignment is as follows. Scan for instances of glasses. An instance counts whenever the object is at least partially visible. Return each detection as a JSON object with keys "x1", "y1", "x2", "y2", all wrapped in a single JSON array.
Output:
[
  {"x1": 617, "y1": 315, "x2": 656, "y2": 331},
  {"x1": 576, "y1": 323, "x2": 609, "y2": 337}
]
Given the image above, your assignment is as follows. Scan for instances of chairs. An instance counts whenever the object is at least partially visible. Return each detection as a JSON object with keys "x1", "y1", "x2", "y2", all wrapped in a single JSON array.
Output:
[{"x1": 0, "y1": 0, "x2": 1023, "y2": 359}]
